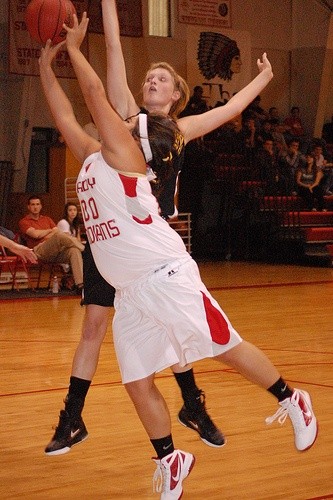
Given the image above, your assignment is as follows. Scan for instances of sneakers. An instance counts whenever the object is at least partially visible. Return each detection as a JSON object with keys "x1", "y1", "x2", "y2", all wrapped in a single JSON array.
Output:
[
  {"x1": 45, "y1": 410, "x2": 88, "y2": 455},
  {"x1": 265, "y1": 387, "x2": 318, "y2": 451},
  {"x1": 151, "y1": 447, "x2": 195, "y2": 500},
  {"x1": 177, "y1": 389, "x2": 227, "y2": 447}
]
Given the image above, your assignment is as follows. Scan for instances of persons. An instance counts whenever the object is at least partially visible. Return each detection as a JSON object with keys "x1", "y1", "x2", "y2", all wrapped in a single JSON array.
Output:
[
  {"x1": 185, "y1": 85, "x2": 333, "y2": 213},
  {"x1": 44, "y1": 0, "x2": 227, "y2": 456},
  {"x1": 39, "y1": 12, "x2": 319, "y2": 500},
  {"x1": 0, "y1": 196, "x2": 86, "y2": 288}
]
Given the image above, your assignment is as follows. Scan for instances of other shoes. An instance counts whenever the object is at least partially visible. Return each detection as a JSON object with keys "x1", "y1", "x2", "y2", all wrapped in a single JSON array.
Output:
[
  {"x1": 322, "y1": 208, "x2": 327, "y2": 212},
  {"x1": 313, "y1": 207, "x2": 317, "y2": 212}
]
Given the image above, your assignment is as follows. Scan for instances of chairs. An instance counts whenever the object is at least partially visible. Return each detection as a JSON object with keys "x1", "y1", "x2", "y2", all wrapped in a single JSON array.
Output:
[
  {"x1": 11, "y1": 231, "x2": 69, "y2": 292},
  {"x1": 0, "y1": 227, "x2": 20, "y2": 296}
]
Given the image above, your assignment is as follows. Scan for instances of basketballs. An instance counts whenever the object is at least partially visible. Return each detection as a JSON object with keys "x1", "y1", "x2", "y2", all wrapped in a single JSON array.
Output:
[{"x1": 23, "y1": 0, "x2": 77, "y2": 47}]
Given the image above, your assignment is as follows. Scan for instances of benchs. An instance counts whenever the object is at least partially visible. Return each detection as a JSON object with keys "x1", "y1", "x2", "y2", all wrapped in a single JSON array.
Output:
[{"x1": 207, "y1": 141, "x2": 333, "y2": 260}]
